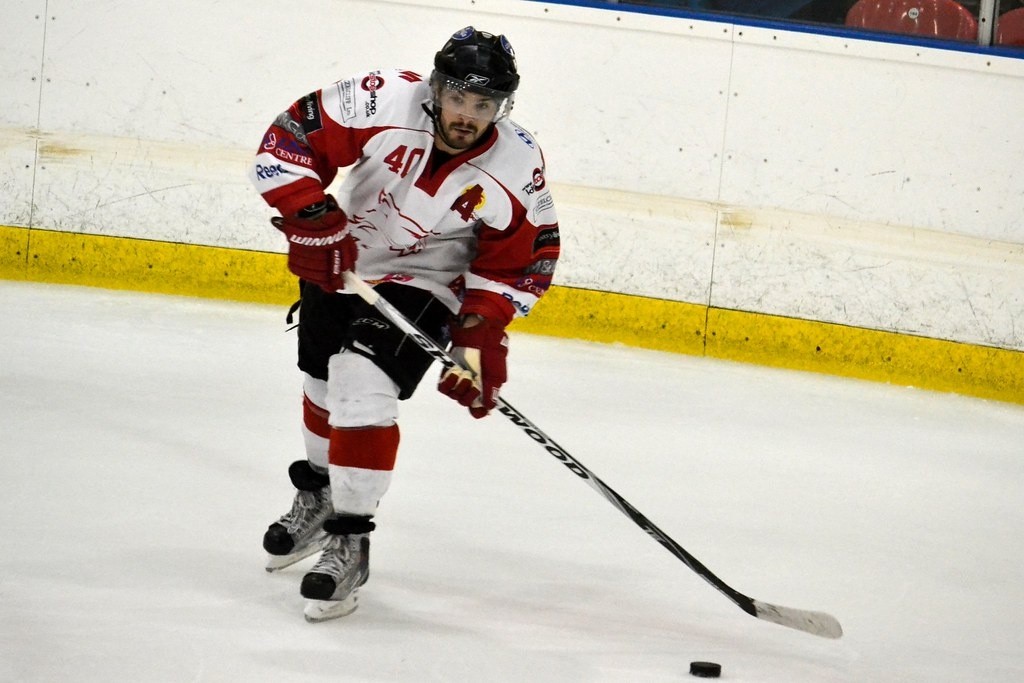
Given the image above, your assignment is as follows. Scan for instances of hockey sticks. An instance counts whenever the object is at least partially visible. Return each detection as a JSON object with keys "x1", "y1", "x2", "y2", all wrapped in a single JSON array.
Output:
[{"x1": 339, "y1": 267, "x2": 846, "y2": 642}]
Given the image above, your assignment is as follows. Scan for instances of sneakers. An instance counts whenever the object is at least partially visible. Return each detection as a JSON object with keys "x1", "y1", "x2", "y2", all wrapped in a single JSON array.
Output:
[
  {"x1": 262, "y1": 460, "x2": 334, "y2": 572},
  {"x1": 300, "y1": 511, "x2": 376, "y2": 623}
]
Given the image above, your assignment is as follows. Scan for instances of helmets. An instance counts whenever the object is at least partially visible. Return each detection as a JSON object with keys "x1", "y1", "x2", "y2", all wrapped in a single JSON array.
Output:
[{"x1": 433, "y1": 26, "x2": 521, "y2": 99}]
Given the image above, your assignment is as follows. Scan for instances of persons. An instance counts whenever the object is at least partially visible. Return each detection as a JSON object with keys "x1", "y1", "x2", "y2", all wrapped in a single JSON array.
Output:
[{"x1": 243, "y1": 23, "x2": 565, "y2": 625}]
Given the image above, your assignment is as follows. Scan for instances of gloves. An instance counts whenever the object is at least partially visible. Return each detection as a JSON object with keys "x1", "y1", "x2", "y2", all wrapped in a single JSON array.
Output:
[
  {"x1": 271, "y1": 209, "x2": 358, "y2": 295},
  {"x1": 438, "y1": 289, "x2": 517, "y2": 419}
]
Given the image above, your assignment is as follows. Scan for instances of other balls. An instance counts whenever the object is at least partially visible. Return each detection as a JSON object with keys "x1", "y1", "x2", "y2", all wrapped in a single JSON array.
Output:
[{"x1": 689, "y1": 661, "x2": 723, "y2": 680}]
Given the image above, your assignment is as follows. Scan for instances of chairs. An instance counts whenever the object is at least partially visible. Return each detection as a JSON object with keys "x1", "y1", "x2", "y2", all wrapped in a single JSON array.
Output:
[{"x1": 846, "y1": 0, "x2": 1024, "y2": 50}]
